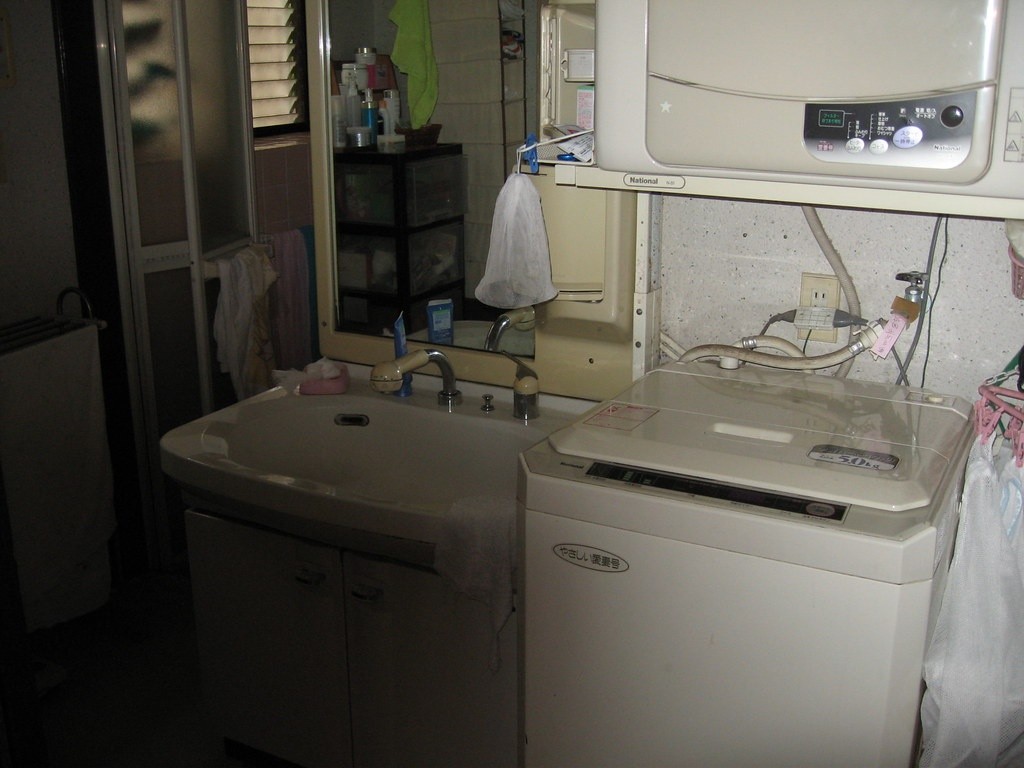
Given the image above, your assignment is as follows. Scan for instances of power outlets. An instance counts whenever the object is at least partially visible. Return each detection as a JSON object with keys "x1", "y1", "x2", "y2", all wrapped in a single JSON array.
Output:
[{"x1": 794, "y1": 273, "x2": 841, "y2": 345}]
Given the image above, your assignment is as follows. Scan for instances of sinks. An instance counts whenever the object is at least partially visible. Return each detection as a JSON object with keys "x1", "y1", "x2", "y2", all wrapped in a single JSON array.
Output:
[{"x1": 159, "y1": 369, "x2": 580, "y2": 571}]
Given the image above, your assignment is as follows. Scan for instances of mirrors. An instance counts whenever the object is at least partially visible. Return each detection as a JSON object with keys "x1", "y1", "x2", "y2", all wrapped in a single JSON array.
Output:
[{"x1": 303, "y1": 0, "x2": 535, "y2": 390}]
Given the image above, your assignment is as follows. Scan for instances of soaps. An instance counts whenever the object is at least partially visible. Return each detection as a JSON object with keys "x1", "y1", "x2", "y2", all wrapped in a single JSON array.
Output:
[{"x1": 304, "y1": 356, "x2": 339, "y2": 380}]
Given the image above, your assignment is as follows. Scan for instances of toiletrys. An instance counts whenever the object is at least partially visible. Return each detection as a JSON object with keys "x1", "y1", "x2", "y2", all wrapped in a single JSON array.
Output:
[
  {"x1": 426, "y1": 298, "x2": 457, "y2": 347},
  {"x1": 331, "y1": 44, "x2": 411, "y2": 156},
  {"x1": 389, "y1": 311, "x2": 415, "y2": 398}
]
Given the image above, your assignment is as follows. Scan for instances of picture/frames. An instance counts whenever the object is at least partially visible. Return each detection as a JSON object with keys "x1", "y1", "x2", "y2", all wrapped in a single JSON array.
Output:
[{"x1": 371, "y1": 54, "x2": 404, "y2": 118}]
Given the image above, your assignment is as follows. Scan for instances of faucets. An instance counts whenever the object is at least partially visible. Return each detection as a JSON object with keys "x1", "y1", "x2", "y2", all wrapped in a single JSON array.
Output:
[
  {"x1": 367, "y1": 347, "x2": 467, "y2": 406},
  {"x1": 891, "y1": 270, "x2": 934, "y2": 326},
  {"x1": 483, "y1": 304, "x2": 538, "y2": 352}
]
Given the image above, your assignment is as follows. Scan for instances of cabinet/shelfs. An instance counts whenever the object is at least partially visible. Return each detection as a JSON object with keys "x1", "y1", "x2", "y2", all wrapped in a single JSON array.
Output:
[{"x1": 333, "y1": 145, "x2": 467, "y2": 334}]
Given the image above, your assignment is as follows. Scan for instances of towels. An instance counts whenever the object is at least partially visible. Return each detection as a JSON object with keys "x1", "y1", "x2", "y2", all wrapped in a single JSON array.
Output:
[
  {"x1": 386, "y1": 0, "x2": 442, "y2": 132},
  {"x1": 499, "y1": 1, "x2": 527, "y2": 21},
  {"x1": 215, "y1": 222, "x2": 318, "y2": 406}
]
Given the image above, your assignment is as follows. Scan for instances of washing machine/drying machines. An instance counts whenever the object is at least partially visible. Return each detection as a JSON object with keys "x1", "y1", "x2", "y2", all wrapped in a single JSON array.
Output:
[{"x1": 507, "y1": 355, "x2": 979, "y2": 768}]
